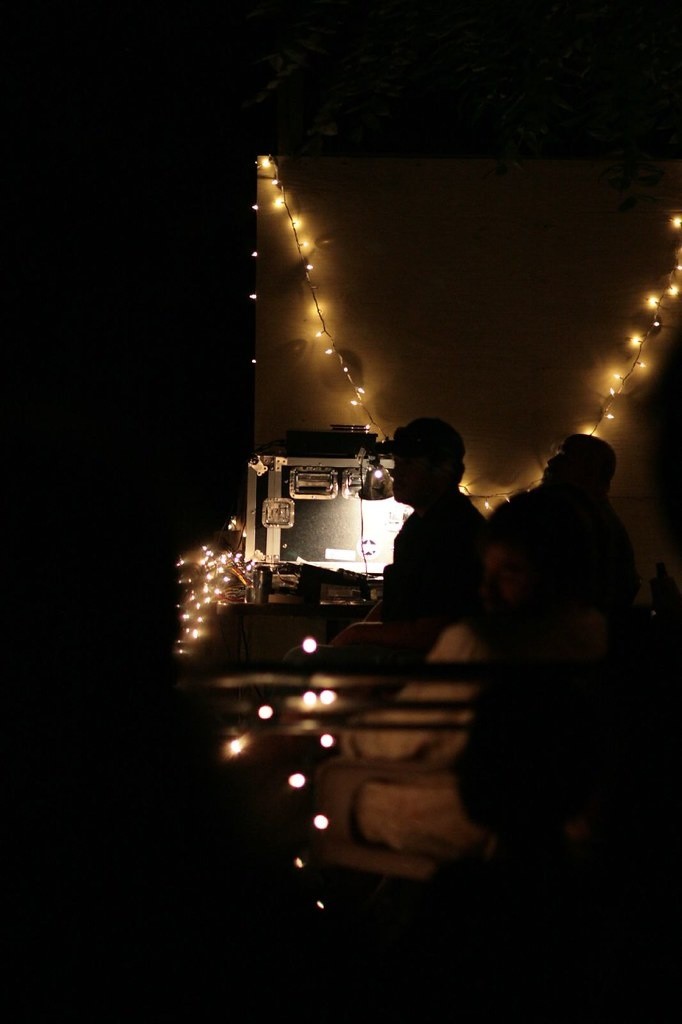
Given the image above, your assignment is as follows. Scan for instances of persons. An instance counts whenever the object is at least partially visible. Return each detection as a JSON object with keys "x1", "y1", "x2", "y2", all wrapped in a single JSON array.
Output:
[{"x1": 326, "y1": 416, "x2": 641, "y2": 646}]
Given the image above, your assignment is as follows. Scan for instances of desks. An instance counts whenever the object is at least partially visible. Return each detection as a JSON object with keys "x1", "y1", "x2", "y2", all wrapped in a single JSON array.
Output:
[{"x1": 216, "y1": 600, "x2": 375, "y2": 645}]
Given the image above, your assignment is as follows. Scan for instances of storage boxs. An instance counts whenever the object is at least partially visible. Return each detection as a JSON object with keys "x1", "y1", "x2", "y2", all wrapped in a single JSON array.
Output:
[
  {"x1": 245, "y1": 453, "x2": 414, "y2": 568},
  {"x1": 285, "y1": 428, "x2": 378, "y2": 458}
]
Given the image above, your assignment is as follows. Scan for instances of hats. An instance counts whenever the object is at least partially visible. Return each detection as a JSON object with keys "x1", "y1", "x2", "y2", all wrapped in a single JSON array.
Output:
[{"x1": 374, "y1": 418, "x2": 466, "y2": 463}]
